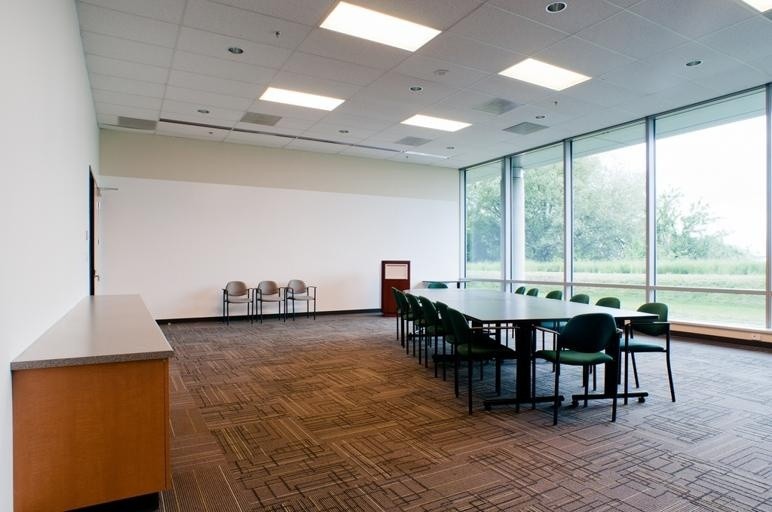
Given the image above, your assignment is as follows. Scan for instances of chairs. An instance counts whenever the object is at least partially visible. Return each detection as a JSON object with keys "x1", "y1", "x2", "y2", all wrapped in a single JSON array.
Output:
[
  {"x1": 620, "y1": 302, "x2": 675, "y2": 401},
  {"x1": 596, "y1": 298, "x2": 620, "y2": 308},
  {"x1": 448, "y1": 309, "x2": 516, "y2": 416},
  {"x1": 527, "y1": 287, "x2": 538, "y2": 296},
  {"x1": 221, "y1": 280, "x2": 255, "y2": 326},
  {"x1": 436, "y1": 302, "x2": 455, "y2": 381},
  {"x1": 286, "y1": 279, "x2": 317, "y2": 322},
  {"x1": 393, "y1": 287, "x2": 413, "y2": 354},
  {"x1": 570, "y1": 294, "x2": 589, "y2": 304},
  {"x1": 429, "y1": 283, "x2": 448, "y2": 289},
  {"x1": 255, "y1": 280, "x2": 287, "y2": 325},
  {"x1": 516, "y1": 287, "x2": 525, "y2": 294},
  {"x1": 532, "y1": 313, "x2": 618, "y2": 425},
  {"x1": 545, "y1": 291, "x2": 562, "y2": 300},
  {"x1": 405, "y1": 293, "x2": 423, "y2": 364},
  {"x1": 419, "y1": 296, "x2": 442, "y2": 378}
]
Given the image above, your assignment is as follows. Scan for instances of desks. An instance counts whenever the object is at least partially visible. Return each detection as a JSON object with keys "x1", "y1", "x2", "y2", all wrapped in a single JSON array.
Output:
[{"x1": 402, "y1": 289, "x2": 660, "y2": 407}]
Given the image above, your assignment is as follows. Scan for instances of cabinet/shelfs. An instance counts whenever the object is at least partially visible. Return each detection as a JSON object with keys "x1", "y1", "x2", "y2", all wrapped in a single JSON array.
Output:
[{"x1": 9, "y1": 294, "x2": 176, "y2": 512}]
